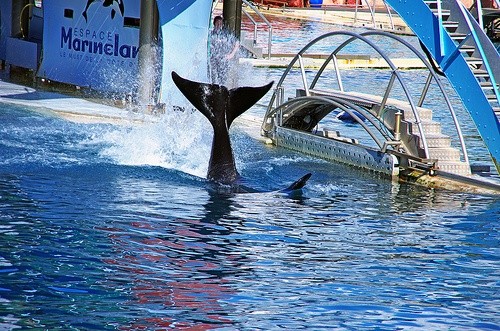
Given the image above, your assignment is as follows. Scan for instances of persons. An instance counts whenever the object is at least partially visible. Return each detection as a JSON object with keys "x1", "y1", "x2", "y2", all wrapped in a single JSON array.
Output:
[{"x1": 208, "y1": 16, "x2": 239, "y2": 88}]
